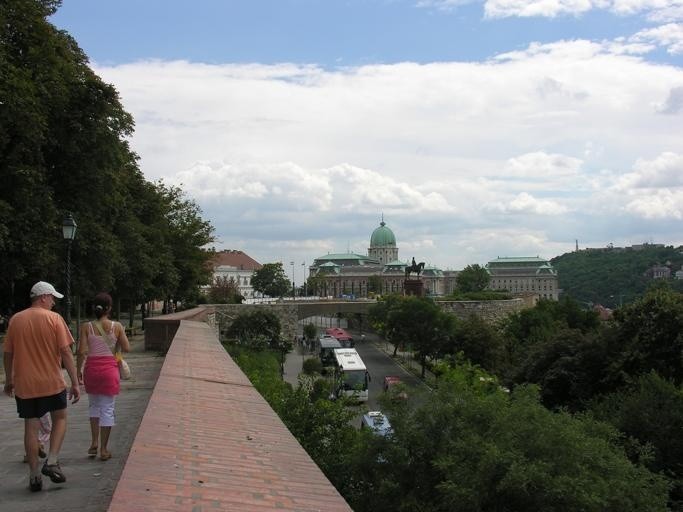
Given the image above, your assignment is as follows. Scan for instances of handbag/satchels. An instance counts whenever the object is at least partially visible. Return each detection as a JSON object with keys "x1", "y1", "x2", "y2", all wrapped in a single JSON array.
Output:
[{"x1": 116, "y1": 353, "x2": 130, "y2": 380}]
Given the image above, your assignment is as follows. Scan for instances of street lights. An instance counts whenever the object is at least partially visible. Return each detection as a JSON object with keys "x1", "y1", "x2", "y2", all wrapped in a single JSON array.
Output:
[
  {"x1": 60, "y1": 211, "x2": 77, "y2": 335},
  {"x1": 289, "y1": 262, "x2": 295, "y2": 300},
  {"x1": 301, "y1": 262, "x2": 306, "y2": 298}
]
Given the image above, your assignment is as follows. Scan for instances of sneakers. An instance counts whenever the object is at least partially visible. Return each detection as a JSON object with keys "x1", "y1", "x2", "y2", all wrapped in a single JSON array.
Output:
[
  {"x1": 100, "y1": 451, "x2": 111, "y2": 460},
  {"x1": 22, "y1": 441, "x2": 48, "y2": 463},
  {"x1": 28, "y1": 478, "x2": 42, "y2": 492},
  {"x1": 87, "y1": 447, "x2": 97, "y2": 455},
  {"x1": 41, "y1": 459, "x2": 65, "y2": 482}
]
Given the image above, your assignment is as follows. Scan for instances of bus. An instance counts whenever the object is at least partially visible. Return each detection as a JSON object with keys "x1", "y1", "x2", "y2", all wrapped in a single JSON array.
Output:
[
  {"x1": 315, "y1": 325, "x2": 372, "y2": 404},
  {"x1": 361, "y1": 409, "x2": 396, "y2": 464}
]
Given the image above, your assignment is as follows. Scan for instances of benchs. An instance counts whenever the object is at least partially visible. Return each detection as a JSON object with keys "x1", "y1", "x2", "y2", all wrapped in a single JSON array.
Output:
[{"x1": 124, "y1": 325, "x2": 139, "y2": 337}]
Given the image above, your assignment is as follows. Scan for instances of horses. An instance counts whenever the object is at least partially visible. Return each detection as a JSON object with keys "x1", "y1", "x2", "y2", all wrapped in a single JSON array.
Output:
[{"x1": 404, "y1": 262, "x2": 425, "y2": 280}]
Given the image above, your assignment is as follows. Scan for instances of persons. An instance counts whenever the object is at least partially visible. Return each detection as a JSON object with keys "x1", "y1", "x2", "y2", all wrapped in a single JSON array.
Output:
[
  {"x1": 76, "y1": 292, "x2": 130, "y2": 461},
  {"x1": 167, "y1": 299, "x2": 176, "y2": 314},
  {"x1": 2, "y1": 280, "x2": 81, "y2": 493},
  {"x1": 293, "y1": 336, "x2": 311, "y2": 353},
  {"x1": 21, "y1": 408, "x2": 51, "y2": 462}
]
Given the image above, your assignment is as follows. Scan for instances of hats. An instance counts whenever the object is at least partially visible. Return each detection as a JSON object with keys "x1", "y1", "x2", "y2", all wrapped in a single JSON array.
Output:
[{"x1": 29, "y1": 280, "x2": 64, "y2": 299}]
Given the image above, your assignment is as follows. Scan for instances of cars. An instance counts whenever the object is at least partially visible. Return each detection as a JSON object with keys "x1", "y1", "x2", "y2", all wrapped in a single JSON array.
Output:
[{"x1": 384, "y1": 375, "x2": 408, "y2": 404}]
